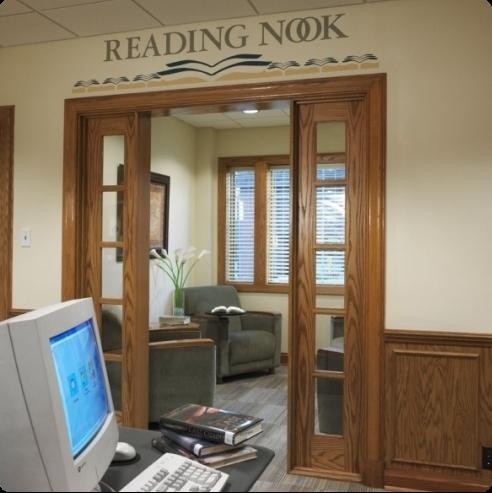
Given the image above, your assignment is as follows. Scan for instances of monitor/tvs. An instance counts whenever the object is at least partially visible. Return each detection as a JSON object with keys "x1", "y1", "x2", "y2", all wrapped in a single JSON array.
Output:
[{"x1": 0, "y1": 296, "x2": 119, "y2": 492}]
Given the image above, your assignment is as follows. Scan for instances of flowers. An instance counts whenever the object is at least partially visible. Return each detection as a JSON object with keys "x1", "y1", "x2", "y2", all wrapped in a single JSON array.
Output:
[{"x1": 149, "y1": 245, "x2": 211, "y2": 288}]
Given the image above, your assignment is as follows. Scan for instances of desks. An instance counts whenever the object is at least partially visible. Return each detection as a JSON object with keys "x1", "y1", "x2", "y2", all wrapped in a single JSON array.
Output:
[{"x1": 100, "y1": 429, "x2": 275, "y2": 493}]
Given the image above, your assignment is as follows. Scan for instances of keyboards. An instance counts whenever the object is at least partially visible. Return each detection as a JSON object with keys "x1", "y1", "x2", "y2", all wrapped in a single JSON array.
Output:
[{"x1": 119, "y1": 452, "x2": 230, "y2": 493}]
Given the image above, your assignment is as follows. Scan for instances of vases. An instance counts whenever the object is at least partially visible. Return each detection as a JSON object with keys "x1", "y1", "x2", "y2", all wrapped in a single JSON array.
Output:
[{"x1": 171, "y1": 282, "x2": 185, "y2": 317}]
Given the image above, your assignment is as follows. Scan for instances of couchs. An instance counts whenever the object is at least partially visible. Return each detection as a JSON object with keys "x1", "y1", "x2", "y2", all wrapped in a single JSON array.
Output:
[
  {"x1": 101, "y1": 307, "x2": 217, "y2": 430},
  {"x1": 176, "y1": 285, "x2": 282, "y2": 381},
  {"x1": 317, "y1": 314, "x2": 345, "y2": 435}
]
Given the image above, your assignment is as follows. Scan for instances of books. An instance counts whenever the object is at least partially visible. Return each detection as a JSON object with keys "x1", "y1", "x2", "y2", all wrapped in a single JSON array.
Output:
[
  {"x1": 159, "y1": 314, "x2": 191, "y2": 325},
  {"x1": 211, "y1": 304, "x2": 244, "y2": 314},
  {"x1": 151, "y1": 401, "x2": 264, "y2": 471}
]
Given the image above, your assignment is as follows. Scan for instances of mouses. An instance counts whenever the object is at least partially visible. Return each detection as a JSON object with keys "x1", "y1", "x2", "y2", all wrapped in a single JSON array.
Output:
[{"x1": 112, "y1": 441, "x2": 137, "y2": 461}]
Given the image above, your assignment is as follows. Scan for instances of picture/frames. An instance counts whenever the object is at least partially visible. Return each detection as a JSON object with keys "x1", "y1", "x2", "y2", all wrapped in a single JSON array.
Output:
[{"x1": 116, "y1": 164, "x2": 170, "y2": 262}]
